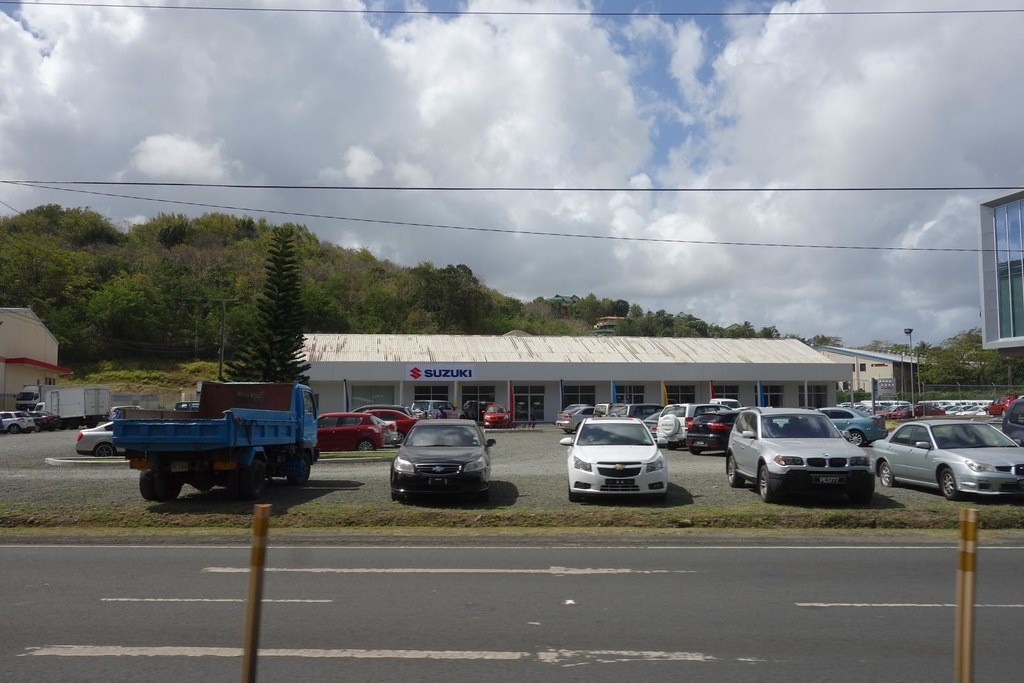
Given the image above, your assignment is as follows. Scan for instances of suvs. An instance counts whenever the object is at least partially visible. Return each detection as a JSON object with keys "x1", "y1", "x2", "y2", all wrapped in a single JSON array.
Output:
[
  {"x1": 726, "y1": 406, "x2": 874, "y2": 502},
  {"x1": 313, "y1": 405, "x2": 421, "y2": 451},
  {"x1": 657, "y1": 403, "x2": 742, "y2": 455},
  {"x1": 1001, "y1": 395, "x2": 1024, "y2": 446},
  {"x1": 592, "y1": 401, "x2": 665, "y2": 420}
]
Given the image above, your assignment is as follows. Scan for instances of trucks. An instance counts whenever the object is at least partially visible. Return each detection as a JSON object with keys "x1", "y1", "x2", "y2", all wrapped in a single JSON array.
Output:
[
  {"x1": 13, "y1": 384, "x2": 112, "y2": 430},
  {"x1": 112, "y1": 379, "x2": 321, "y2": 503}
]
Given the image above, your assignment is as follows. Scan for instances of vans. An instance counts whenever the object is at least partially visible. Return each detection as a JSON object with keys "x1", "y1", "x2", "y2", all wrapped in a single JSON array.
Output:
[{"x1": 709, "y1": 397, "x2": 743, "y2": 410}]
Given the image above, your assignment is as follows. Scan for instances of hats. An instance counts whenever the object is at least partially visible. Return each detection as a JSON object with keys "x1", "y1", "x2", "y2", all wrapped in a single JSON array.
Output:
[{"x1": 439, "y1": 406, "x2": 443, "y2": 409}]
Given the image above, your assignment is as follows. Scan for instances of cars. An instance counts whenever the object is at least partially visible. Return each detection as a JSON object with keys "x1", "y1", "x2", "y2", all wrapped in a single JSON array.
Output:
[
  {"x1": 389, "y1": 419, "x2": 496, "y2": 505},
  {"x1": 815, "y1": 406, "x2": 888, "y2": 446},
  {"x1": 639, "y1": 412, "x2": 662, "y2": 442},
  {"x1": 558, "y1": 417, "x2": 669, "y2": 500},
  {"x1": 870, "y1": 419, "x2": 1024, "y2": 501},
  {"x1": 0, "y1": 410, "x2": 64, "y2": 435},
  {"x1": 75, "y1": 421, "x2": 128, "y2": 458},
  {"x1": 874, "y1": 403, "x2": 946, "y2": 419},
  {"x1": 482, "y1": 405, "x2": 510, "y2": 428},
  {"x1": 411, "y1": 399, "x2": 467, "y2": 420},
  {"x1": 555, "y1": 403, "x2": 596, "y2": 434},
  {"x1": 938, "y1": 395, "x2": 1015, "y2": 417}
]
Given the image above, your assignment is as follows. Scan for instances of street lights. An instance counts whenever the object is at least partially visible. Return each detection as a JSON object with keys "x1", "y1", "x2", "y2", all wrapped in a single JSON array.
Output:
[{"x1": 904, "y1": 328, "x2": 914, "y2": 417}]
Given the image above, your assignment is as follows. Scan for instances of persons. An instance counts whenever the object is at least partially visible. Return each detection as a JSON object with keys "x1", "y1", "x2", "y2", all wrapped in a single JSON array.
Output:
[{"x1": 434, "y1": 406, "x2": 447, "y2": 419}]
